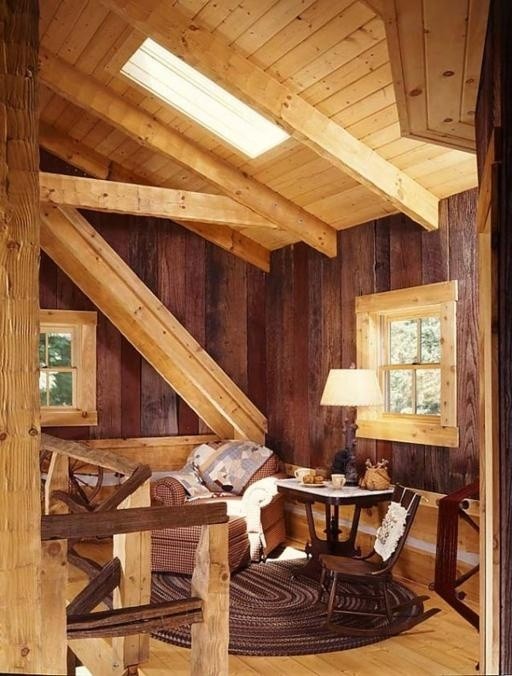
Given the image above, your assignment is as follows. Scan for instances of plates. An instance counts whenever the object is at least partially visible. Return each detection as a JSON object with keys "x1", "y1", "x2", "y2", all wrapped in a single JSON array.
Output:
[{"x1": 299, "y1": 481, "x2": 328, "y2": 488}]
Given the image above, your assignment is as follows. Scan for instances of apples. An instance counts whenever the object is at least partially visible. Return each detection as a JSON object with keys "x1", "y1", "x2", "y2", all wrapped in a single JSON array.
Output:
[
  {"x1": 314, "y1": 476, "x2": 324, "y2": 484},
  {"x1": 302, "y1": 474, "x2": 314, "y2": 483}
]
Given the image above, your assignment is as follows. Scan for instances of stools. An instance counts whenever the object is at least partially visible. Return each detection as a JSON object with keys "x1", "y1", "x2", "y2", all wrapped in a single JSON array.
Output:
[{"x1": 150, "y1": 515, "x2": 252, "y2": 578}]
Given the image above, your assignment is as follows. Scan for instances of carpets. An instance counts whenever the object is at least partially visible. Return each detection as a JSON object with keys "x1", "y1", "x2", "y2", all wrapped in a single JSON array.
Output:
[{"x1": 150, "y1": 544, "x2": 424, "y2": 658}]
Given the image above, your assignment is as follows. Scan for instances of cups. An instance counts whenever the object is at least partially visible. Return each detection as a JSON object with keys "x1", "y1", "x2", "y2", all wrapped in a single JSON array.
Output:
[
  {"x1": 331, "y1": 474, "x2": 346, "y2": 490},
  {"x1": 294, "y1": 468, "x2": 311, "y2": 482}
]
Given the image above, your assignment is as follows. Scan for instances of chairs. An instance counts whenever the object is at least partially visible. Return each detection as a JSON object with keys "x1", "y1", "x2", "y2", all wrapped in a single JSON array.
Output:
[
  {"x1": 151, "y1": 440, "x2": 286, "y2": 563},
  {"x1": 315, "y1": 482, "x2": 441, "y2": 639}
]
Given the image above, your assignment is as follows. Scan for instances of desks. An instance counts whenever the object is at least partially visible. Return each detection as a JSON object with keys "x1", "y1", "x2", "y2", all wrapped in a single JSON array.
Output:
[{"x1": 273, "y1": 475, "x2": 398, "y2": 582}]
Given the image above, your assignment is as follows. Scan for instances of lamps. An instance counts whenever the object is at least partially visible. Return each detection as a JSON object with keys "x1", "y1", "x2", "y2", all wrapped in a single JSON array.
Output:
[{"x1": 319, "y1": 361, "x2": 386, "y2": 485}]
